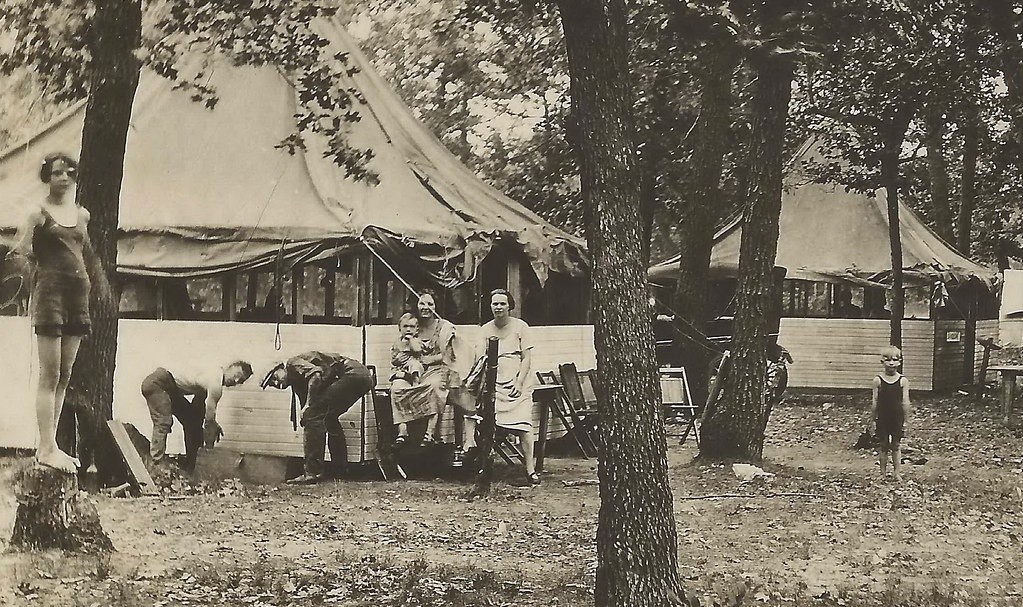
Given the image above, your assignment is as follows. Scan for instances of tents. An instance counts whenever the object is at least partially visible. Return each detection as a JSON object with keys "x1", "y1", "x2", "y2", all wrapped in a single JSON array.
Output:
[
  {"x1": 0, "y1": 1, "x2": 595, "y2": 465},
  {"x1": 646, "y1": 115, "x2": 1000, "y2": 400}
]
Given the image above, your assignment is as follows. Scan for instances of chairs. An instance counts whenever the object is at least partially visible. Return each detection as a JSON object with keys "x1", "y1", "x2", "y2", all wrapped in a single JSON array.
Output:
[
  {"x1": 659, "y1": 365, "x2": 700, "y2": 450},
  {"x1": 536, "y1": 363, "x2": 600, "y2": 459}
]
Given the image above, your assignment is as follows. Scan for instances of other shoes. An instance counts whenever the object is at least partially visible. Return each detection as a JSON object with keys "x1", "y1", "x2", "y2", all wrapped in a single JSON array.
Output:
[
  {"x1": 394, "y1": 432, "x2": 410, "y2": 444},
  {"x1": 419, "y1": 432, "x2": 435, "y2": 448},
  {"x1": 286, "y1": 465, "x2": 324, "y2": 485},
  {"x1": 525, "y1": 466, "x2": 541, "y2": 484},
  {"x1": 389, "y1": 371, "x2": 399, "y2": 381}
]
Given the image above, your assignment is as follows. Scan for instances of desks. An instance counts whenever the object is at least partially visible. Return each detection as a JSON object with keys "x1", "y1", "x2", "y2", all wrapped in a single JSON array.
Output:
[
  {"x1": 376, "y1": 385, "x2": 566, "y2": 481},
  {"x1": 986, "y1": 365, "x2": 1023, "y2": 414}
]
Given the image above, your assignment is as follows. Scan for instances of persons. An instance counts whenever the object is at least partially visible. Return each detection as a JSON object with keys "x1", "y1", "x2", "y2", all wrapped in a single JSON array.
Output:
[
  {"x1": 459, "y1": 289, "x2": 540, "y2": 486},
  {"x1": 389, "y1": 289, "x2": 459, "y2": 448},
  {"x1": 141, "y1": 361, "x2": 252, "y2": 472},
  {"x1": 835, "y1": 290, "x2": 861, "y2": 317},
  {"x1": 871, "y1": 346, "x2": 910, "y2": 481},
  {"x1": 259, "y1": 350, "x2": 374, "y2": 484},
  {"x1": 15, "y1": 153, "x2": 109, "y2": 473}
]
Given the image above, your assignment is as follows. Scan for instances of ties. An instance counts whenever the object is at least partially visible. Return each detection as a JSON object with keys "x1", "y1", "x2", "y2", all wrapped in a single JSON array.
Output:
[{"x1": 291, "y1": 386, "x2": 296, "y2": 431}]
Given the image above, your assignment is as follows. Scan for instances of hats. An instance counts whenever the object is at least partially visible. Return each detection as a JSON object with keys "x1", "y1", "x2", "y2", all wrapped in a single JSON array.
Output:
[{"x1": 258, "y1": 358, "x2": 285, "y2": 390}]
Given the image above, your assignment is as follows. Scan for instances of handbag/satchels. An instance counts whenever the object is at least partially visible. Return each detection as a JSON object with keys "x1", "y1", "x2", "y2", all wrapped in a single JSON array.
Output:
[{"x1": 447, "y1": 354, "x2": 488, "y2": 415}]
